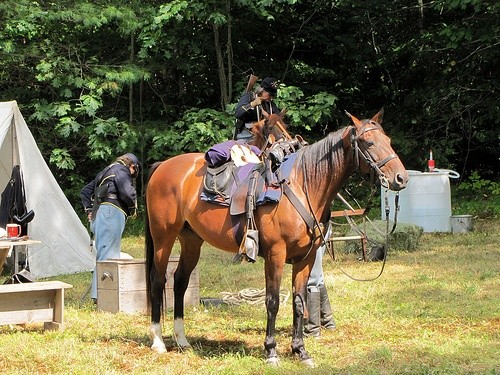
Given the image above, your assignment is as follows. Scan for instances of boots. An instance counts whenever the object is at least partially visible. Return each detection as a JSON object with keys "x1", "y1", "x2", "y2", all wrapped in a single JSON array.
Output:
[
  {"x1": 319, "y1": 286, "x2": 336, "y2": 331},
  {"x1": 303, "y1": 291, "x2": 321, "y2": 338}
]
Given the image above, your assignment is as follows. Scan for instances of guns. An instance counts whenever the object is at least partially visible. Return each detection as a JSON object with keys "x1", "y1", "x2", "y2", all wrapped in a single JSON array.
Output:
[
  {"x1": 89, "y1": 183, "x2": 109, "y2": 252},
  {"x1": 232, "y1": 74, "x2": 258, "y2": 141}
]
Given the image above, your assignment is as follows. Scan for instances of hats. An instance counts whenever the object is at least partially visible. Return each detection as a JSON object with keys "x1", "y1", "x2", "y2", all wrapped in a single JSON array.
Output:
[
  {"x1": 260, "y1": 77, "x2": 280, "y2": 95},
  {"x1": 126, "y1": 153, "x2": 140, "y2": 178}
]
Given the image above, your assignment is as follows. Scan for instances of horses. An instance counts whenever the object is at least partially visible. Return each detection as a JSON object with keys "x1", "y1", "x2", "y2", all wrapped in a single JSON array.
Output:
[{"x1": 143, "y1": 106, "x2": 409, "y2": 370}]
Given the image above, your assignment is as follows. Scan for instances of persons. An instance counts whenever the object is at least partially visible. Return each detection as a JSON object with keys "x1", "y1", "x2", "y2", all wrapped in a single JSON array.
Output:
[
  {"x1": 303, "y1": 226, "x2": 336, "y2": 336},
  {"x1": 234, "y1": 78, "x2": 279, "y2": 143},
  {"x1": 81, "y1": 153, "x2": 140, "y2": 303}
]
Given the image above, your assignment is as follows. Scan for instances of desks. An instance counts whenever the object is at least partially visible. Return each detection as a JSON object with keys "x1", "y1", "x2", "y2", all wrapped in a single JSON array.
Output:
[{"x1": 0, "y1": 239, "x2": 42, "y2": 274}]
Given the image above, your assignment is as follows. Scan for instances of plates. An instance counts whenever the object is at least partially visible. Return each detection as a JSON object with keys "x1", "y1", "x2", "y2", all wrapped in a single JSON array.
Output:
[{"x1": 2, "y1": 237, "x2": 23, "y2": 241}]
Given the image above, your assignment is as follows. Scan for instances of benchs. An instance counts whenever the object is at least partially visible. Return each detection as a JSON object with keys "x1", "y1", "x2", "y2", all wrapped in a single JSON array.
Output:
[{"x1": 0, "y1": 280, "x2": 74, "y2": 331}]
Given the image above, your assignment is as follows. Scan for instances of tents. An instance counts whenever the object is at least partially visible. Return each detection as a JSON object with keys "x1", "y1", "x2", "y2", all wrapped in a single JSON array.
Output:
[{"x1": 0, "y1": 101, "x2": 95, "y2": 279}]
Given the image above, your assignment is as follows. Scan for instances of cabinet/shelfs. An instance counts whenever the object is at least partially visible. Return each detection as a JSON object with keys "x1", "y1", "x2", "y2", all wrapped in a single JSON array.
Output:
[{"x1": 97, "y1": 258, "x2": 199, "y2": 313}]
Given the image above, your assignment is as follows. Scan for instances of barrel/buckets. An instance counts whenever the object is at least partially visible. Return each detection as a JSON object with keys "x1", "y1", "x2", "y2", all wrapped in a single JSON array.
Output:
[{"x1": 450, "y1": 215, "x2": 474, "y2": 234}]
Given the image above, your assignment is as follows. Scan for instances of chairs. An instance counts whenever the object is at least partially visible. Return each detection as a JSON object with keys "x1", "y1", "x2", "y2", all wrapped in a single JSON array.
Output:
[{"x1": 328, "y1": 209, "x2": 368, "y2": 262}]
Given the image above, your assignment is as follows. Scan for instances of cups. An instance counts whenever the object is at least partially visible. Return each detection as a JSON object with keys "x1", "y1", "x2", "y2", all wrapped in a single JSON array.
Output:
[{"x1": 6, "y1": 223, "x2": 21, "y2": 238}]
